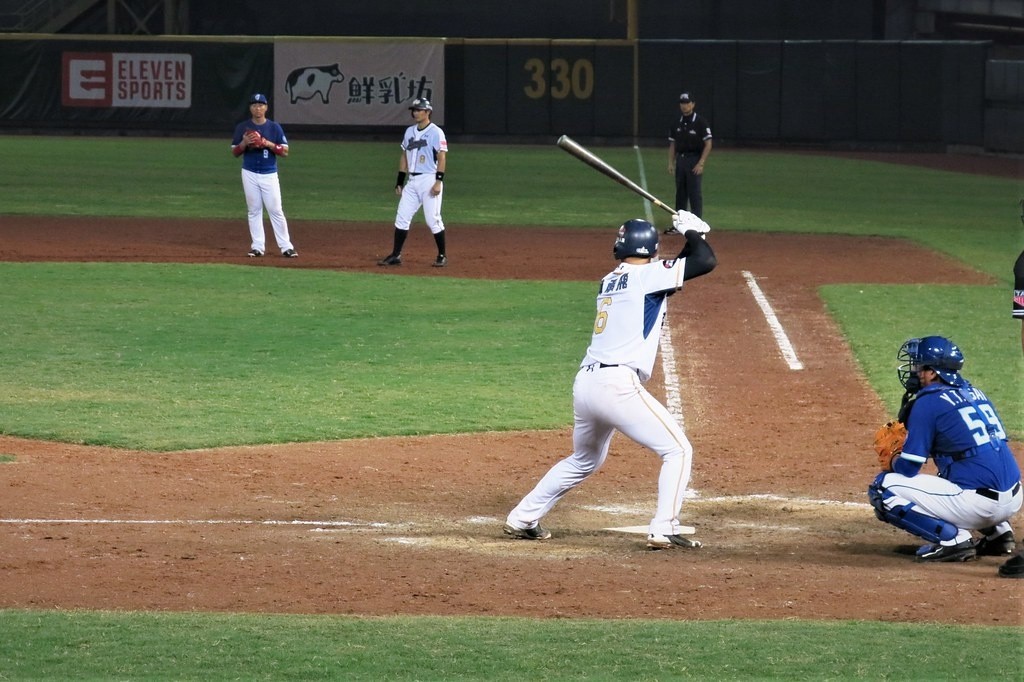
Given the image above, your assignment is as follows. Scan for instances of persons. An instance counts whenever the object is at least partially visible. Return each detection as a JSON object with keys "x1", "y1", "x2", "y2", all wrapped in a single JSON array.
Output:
[
  {"x1": 378, "y1": 98, "x2": 448, "y2": 267},
  {"x1": 868, "y1": 336, "x2": 1024, "y2": 562},
  {"x1": 661, "y1": 93, "x2": 713, "y2": 238},
  {"x1": 1000, "y1": 202, "x2": 1024, "y2": 579},
  {"x1": 504, "y1": 210, "x2": 718, "y2": 548},
  {"x1": 231, "y1": 94, "x2": 299, "y2": 258}
]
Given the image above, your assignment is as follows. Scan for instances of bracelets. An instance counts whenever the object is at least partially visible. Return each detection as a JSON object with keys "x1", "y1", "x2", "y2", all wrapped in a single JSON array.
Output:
[
  {"x1": 233, "y1": 144, "x2": 243, "y2": 157},
  {"x1": 272, "y1": 143, "x2": 283, "y2": 154},
  {"x1": 436, "y1": 172, "x2": 446, "y2": 182}
]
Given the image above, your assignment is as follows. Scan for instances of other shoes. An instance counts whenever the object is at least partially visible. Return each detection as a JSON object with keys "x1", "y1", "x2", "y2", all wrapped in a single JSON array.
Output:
[{"x1": 998, "y1": 555, "x2": 1024, "y2": 579}]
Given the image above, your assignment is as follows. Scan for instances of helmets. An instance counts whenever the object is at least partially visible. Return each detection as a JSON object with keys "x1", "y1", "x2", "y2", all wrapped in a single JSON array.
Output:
[
  {"x1": 614, "y1": 218, "x2": 660, "y2": 259},
  {"x1": 408, "y1": 97, "x2": 434, "y2": 113},
  {"x1": 914, "y1": 336, "x2": 964, "y2": 387}
]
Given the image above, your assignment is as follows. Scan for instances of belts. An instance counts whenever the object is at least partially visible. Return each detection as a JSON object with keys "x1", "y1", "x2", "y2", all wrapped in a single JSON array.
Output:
[
  {"x1": 409, "y1": 172, "x2": 422, "y2": 176},
  {"x1": 976, "y1": 483, "x2": 1021, "y2": 500},
  {"x1": 601, "y1": 363, "x2": 639, "y2": 374}
]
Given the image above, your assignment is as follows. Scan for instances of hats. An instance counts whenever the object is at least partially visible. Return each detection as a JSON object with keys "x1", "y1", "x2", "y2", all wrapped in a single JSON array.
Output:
[
  {"x1": 249, "y1": 94, "x2": 266, "y2": 105},
  {"x1": 678, "y1": 92, "x2": 694, "y2": 104}
]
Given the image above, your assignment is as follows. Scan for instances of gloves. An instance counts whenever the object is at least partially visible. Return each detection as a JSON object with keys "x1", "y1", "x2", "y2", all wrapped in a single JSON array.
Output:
[{"x1": 671, "y1": 210, "x2": 712, "y2": 238}]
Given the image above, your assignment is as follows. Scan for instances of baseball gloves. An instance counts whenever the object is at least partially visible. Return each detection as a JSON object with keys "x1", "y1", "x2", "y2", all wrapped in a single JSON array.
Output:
[
  {"x1": 873, "y1": 416, "x2": 910, "y2": 471},
  {"x1": 246, "y1": 129, "x2": 265, "y2": 149}
]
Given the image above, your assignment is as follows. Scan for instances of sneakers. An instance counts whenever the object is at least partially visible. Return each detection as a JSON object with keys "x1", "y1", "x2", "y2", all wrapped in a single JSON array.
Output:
[
  {"x1": 915, "y1": 536, "x2": 977, "y2": 561},
  {"x1": 646, "y1": 534, "x2": 703, "y2": 548},
  {"x1": 284, "y1": 249, "x2": 298, "y2": 257},
  {"x1": 974, "y1": 529, "x2": 1016, "y2": 555},
  {"x1": 503, "y1": 522, "x2": 552, "y2": 539},
  {"x1": 248, "y1": 249, "x2": 261, "y2": 257},
  {"x1": 665, "y1": 226, "x2": 679, "y2": 236},
  {"x1": 434, "y1": 253, "x2": 447, "y2": 266},
  {"x1": 376, "y1": 253, "x2": 402, "y2": 265}
]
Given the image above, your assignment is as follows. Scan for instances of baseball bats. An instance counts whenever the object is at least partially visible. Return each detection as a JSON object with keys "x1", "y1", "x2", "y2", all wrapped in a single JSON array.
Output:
[{"x1": 556, "y1": 134, "x2": 679, "y2": 217}]
